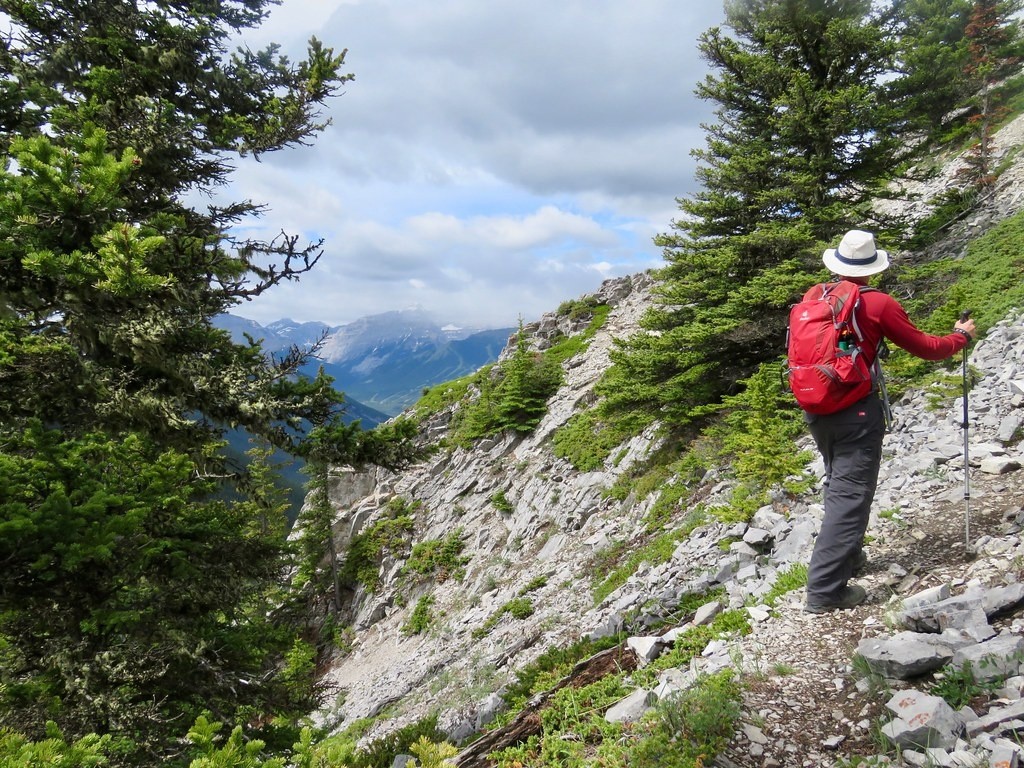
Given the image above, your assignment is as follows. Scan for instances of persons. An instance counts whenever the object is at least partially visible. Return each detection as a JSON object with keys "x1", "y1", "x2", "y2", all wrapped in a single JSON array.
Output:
[{"x1": 786, "y1": 227, "x2": 978, "y2": 614}]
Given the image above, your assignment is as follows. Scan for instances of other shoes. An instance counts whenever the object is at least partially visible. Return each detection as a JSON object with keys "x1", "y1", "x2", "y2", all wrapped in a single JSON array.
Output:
[
  {"x1": 805, "y1": 586, "x2": 866, "y2": 614},
  {"x1": 852, "y1": 550, "x2": 866, "y2": 577}
]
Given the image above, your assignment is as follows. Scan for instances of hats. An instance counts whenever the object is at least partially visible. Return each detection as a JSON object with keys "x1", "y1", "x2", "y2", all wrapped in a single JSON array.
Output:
[{"x1": 822, "y1": 230, "x2": 889, "y2": 277}]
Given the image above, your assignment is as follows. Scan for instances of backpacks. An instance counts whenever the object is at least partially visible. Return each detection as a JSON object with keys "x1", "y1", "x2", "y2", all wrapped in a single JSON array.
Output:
[{"x1": 779, "y1": 280, "x2": 876, "y2": 414}]
